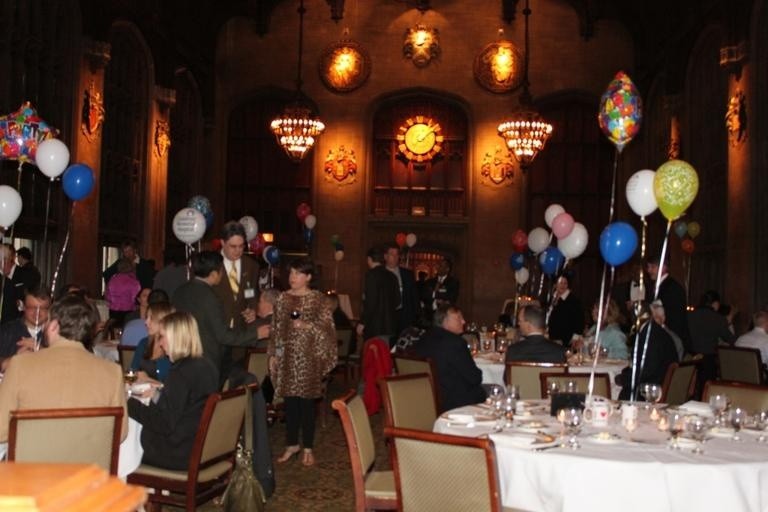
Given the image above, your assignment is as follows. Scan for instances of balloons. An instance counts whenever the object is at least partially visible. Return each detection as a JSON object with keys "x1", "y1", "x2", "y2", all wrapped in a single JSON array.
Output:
[
  {"x1": 0, "y1": 99, "x2": 97, "y2": 229},
  {"x1": 597, "y1": 158, "x2": 705, "y2": 269},
  {"x1": 510, "y1": 202, "x2": 589, "y2": 286},
  {"x1": 296, "y1": 203, "x2": 345, "y2": 262},
  {"x1": 596, "y1": 69, "x2": 644, "y2": 155},
  {"x1": 171, "y1": 195, "x2": 214, "y2": 246}
]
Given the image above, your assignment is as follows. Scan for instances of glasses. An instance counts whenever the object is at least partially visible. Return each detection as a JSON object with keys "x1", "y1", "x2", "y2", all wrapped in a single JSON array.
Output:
[{"x1": 23, "y1": 305, "x2": 49, "y2": 313}]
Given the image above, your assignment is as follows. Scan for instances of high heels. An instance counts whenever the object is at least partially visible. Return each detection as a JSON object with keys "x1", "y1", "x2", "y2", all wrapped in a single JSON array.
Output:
[
  {"x1": 276, "y1": 444, "x2": 301, "y2": 463},
  {"x1": 303, "y1": 450, "x2": 314, "y2": 466}
]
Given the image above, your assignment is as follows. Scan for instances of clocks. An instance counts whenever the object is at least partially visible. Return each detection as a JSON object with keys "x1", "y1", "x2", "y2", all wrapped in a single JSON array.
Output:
[{"x1": 397, "y1": 115, "x2": 444, "y2": 162}]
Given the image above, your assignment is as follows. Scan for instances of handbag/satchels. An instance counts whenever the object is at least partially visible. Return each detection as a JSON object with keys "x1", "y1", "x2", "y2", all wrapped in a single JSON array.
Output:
[{"x1": 218, "y1": 448, "x2": 267, "y2": 512}]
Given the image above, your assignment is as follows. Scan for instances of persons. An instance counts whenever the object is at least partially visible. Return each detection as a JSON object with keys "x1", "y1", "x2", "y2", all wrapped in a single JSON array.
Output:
[
  {"x1": 0, "y1": 221, "x2": 359, "y2": 511},
  {"x1": 348, "y1": 241, "x2": 767, "y2": 418}
]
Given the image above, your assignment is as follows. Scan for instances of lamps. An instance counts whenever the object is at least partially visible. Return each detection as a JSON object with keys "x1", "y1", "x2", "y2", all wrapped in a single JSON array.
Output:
[
  {"x1": 497, "y1": 0, "x2": 553, "y2": 171},
  {"x1": 270, "y1": 0, "x2": 327, "y2": 163}
]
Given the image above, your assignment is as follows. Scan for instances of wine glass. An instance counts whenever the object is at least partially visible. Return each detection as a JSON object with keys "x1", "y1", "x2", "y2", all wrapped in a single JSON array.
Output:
[
  {"x1": 289, "y1": 310, "x2": 299, "y2": 322},
  {"x1": 440, "y1": 380, "x2": 768, "y2": 454},
  {"x1": 497, "y1": 342, "x2": 506, "y2": 363},
  {"x1": 125, "y1": 368, "x2": 137, "y2": 396},
  {"x1": 483, "y1": 340, "x2": 490, "y2": 353}
]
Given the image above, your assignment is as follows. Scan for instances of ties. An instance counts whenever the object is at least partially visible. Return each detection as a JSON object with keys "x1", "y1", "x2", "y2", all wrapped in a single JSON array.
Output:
[{"x1": 227, "y1": 261, "x2": 240, "y2": 302}]
